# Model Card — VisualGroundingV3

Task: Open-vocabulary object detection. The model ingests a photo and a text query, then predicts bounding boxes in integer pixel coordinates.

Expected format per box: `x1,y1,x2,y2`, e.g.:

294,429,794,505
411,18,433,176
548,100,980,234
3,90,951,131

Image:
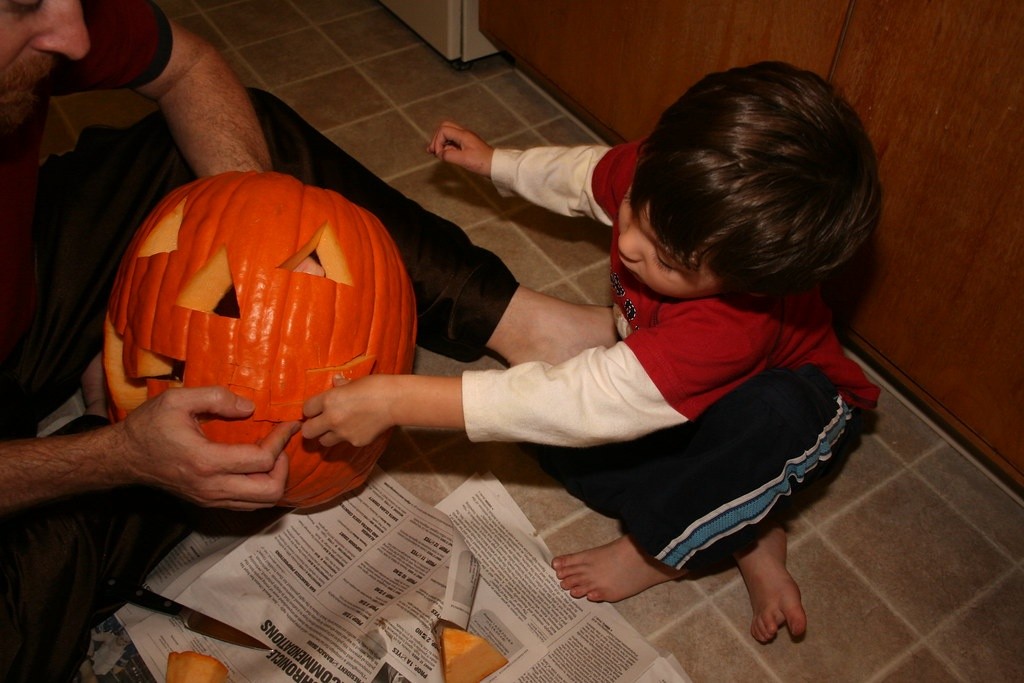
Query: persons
0,0,623,683
301,60,882,645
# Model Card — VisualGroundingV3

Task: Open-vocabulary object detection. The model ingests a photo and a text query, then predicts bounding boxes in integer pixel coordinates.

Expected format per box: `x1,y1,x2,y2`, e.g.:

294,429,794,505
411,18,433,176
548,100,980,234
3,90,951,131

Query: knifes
128,586,275,653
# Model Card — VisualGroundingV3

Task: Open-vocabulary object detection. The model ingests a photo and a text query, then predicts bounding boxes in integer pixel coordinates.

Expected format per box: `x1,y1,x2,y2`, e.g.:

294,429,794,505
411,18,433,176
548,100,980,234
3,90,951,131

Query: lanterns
102,169,419,512
